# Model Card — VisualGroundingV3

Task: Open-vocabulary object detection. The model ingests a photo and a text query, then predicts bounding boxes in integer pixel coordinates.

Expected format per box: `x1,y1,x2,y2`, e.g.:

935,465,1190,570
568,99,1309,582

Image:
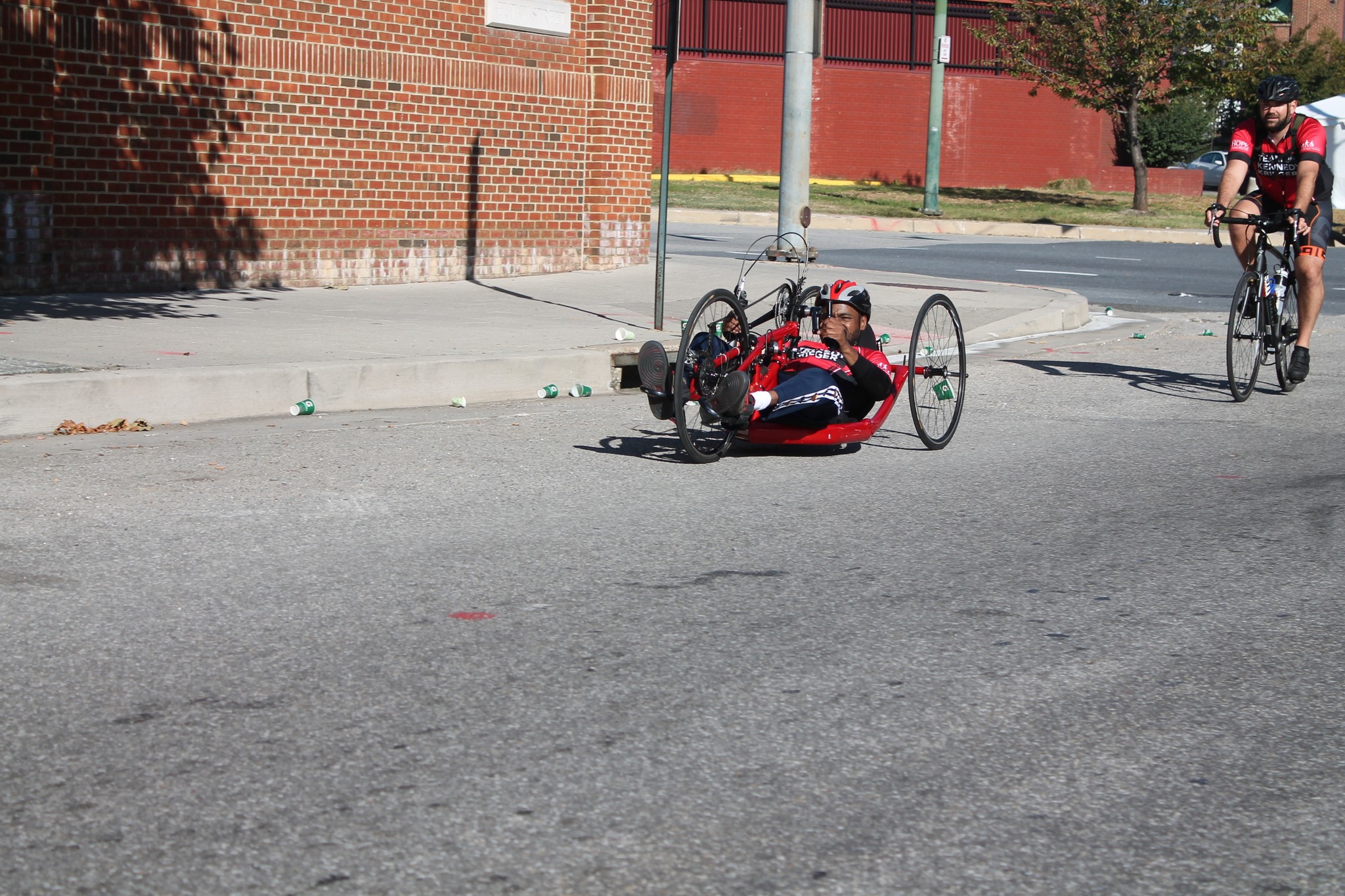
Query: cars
1167,151,1259,195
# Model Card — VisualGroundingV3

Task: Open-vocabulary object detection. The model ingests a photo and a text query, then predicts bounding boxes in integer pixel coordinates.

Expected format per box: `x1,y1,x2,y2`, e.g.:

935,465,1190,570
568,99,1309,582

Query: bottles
1270,263,1291,324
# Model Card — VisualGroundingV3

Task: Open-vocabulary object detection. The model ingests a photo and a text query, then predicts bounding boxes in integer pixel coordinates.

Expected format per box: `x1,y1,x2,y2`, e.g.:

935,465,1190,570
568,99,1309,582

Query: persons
1205,75,1334,380
637,279,893,425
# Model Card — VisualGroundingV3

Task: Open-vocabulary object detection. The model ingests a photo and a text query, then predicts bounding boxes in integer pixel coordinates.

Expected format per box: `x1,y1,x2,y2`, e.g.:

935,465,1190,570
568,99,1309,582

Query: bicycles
1207,204,1301,402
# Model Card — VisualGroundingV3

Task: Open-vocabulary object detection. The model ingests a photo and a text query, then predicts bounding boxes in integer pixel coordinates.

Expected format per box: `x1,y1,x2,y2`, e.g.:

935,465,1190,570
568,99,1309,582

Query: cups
1202,329,1215,335
289,398,315,416
452,396,467,408
536,384,558,399
1131,332,1147,339
715,321,723,338
876,334,890,345
572,383,593,398
933,378,955,401
681,319,688,334
920,347,935,355
1105,306,1114,316
615,328,634,341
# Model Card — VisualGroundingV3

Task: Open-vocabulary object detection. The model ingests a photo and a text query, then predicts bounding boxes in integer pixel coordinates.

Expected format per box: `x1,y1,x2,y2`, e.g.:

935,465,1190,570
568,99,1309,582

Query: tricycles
639,231,968,463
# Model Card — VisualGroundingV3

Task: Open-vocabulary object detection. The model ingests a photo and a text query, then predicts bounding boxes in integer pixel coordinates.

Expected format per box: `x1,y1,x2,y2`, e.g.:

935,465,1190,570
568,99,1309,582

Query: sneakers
1287,349,1310,380
1236,285,1258,318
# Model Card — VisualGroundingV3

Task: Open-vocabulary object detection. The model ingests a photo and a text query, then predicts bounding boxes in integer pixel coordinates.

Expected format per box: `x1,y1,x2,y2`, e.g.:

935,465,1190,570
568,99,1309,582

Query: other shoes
699,371,755,424
638,340,690,420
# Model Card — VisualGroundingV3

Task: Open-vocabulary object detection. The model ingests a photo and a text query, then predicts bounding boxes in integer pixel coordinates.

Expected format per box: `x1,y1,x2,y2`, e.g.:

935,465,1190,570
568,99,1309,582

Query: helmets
1256,76,1299,102
813,279,871,324
